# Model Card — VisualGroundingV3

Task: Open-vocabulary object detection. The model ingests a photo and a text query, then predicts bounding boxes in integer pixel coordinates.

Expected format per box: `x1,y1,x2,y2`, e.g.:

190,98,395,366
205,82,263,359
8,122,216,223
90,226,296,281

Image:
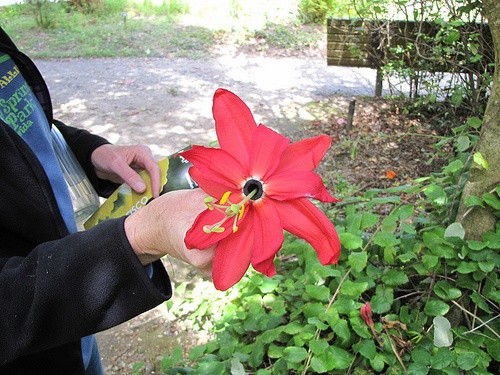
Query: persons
0,24,219,375
